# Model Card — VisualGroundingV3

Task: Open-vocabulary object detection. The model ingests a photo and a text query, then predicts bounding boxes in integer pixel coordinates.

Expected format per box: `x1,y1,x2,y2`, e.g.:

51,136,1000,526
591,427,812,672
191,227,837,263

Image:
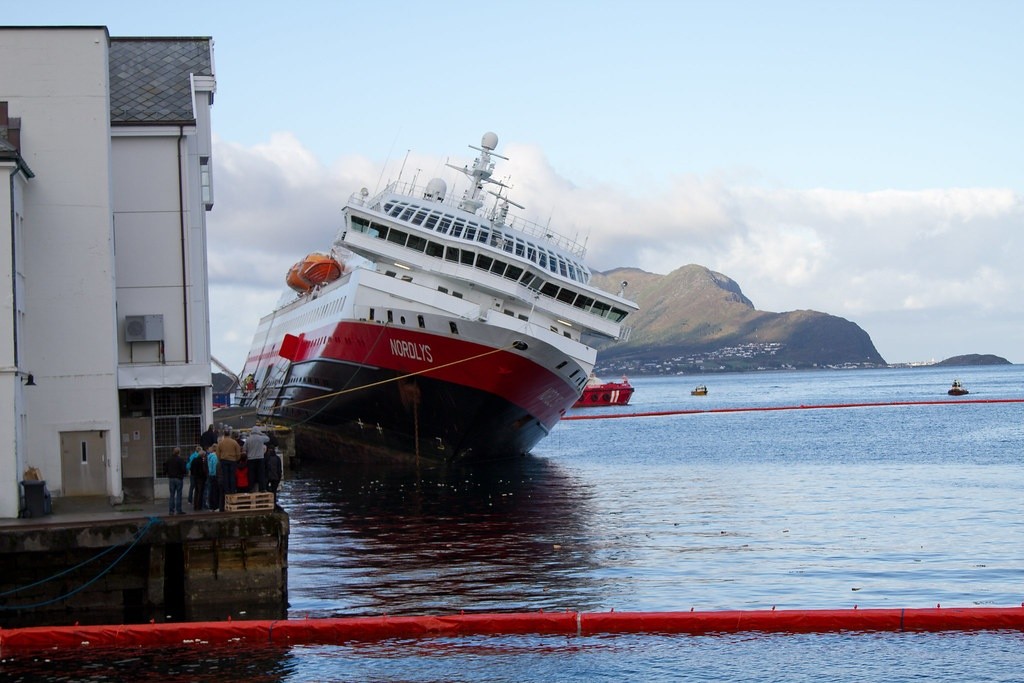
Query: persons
216,431,241,494
201,424,245,451
186,444,220,512
162,448,187,515
243,426,270,493
242,374,254,389
263,448,282,503
236,462,249,494
622,373,628,383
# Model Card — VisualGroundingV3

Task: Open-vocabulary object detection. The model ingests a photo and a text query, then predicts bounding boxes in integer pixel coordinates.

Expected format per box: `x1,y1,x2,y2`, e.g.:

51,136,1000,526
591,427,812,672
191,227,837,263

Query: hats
251,426,261,434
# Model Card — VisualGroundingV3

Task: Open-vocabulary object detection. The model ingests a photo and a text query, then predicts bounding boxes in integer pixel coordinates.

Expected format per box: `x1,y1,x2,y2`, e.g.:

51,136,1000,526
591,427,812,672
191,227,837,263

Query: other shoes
177,512,185,515
170,511,174,515
207,508,219,512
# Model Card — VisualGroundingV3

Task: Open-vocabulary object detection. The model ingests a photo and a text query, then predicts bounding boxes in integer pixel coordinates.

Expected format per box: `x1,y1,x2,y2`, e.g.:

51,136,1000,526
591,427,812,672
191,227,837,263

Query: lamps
21,374,36,386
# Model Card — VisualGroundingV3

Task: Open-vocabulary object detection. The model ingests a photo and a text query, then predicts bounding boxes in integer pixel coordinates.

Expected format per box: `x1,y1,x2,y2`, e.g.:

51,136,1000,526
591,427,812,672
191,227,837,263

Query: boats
690,385,708,395
297,254,343,286
571,374,634,407
285,262,319,293
230,130,640,478
947,380,970,395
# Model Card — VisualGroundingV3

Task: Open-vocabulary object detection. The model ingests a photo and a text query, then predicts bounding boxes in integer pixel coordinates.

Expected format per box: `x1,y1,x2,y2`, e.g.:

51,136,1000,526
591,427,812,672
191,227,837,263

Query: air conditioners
124,313,165,342
